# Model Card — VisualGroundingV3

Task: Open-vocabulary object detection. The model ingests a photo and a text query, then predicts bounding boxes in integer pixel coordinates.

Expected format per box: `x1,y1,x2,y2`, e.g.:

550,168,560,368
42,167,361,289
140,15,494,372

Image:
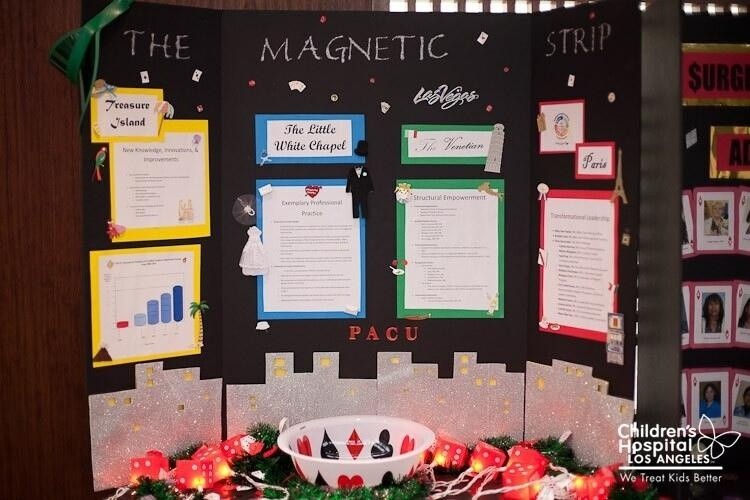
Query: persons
701,293,725,333
733,386,750,418
704,201,729,237
699,383,721,418
738,296,750,329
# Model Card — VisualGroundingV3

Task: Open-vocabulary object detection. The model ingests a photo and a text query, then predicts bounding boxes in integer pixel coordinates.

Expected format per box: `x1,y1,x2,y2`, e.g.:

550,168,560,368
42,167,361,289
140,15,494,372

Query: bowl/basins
273,413,438,491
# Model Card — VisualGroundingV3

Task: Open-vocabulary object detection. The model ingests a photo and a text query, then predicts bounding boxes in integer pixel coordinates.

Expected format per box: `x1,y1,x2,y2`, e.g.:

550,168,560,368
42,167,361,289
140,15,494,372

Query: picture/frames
536,99,586,156
